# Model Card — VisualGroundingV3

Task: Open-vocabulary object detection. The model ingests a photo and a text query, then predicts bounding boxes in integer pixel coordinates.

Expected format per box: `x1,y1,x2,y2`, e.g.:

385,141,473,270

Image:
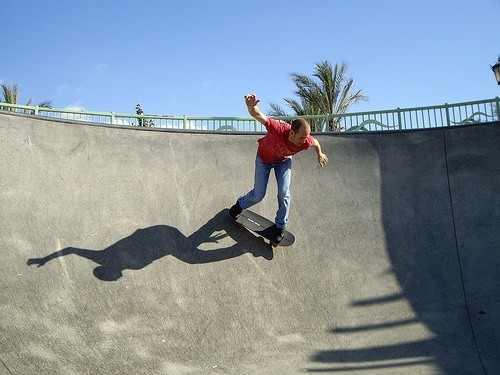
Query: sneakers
273,227,286,244
233,198,243,219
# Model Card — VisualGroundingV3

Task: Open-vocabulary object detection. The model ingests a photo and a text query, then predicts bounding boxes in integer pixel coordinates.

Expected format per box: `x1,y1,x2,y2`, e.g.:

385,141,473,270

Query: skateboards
231,209,295,247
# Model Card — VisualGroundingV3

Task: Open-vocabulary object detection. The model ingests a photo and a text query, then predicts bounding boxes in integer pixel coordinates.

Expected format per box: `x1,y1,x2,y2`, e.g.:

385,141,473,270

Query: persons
229,92,329,244
